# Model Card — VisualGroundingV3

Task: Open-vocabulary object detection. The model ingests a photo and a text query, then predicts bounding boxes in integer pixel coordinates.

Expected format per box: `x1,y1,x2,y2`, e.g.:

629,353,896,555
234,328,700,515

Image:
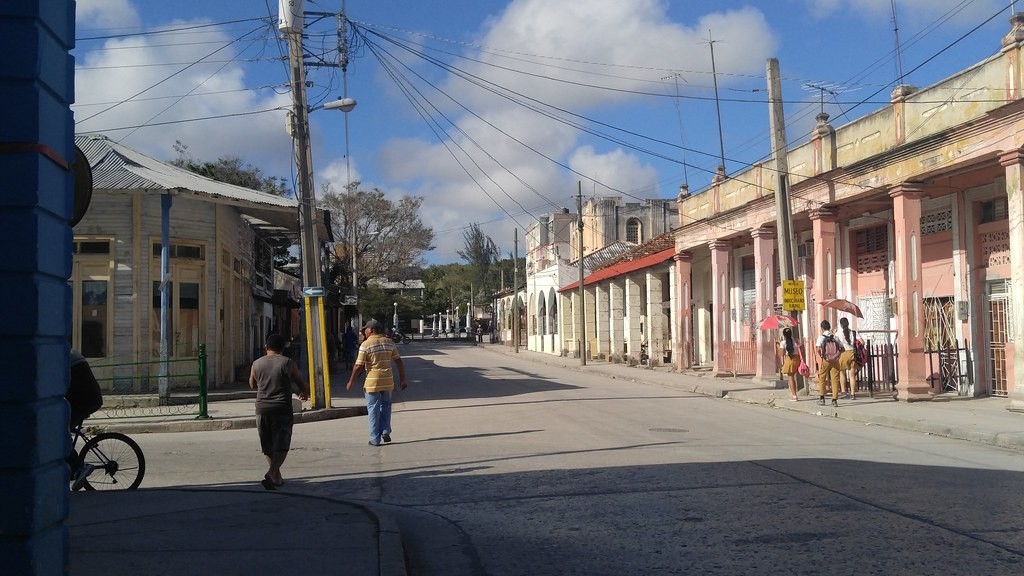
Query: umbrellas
818,296,864,328
751,314,800,330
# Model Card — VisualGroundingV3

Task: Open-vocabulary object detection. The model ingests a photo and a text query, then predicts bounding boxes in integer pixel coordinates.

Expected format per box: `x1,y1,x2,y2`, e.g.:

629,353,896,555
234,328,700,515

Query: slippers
274,478,283,486
261,478,277,490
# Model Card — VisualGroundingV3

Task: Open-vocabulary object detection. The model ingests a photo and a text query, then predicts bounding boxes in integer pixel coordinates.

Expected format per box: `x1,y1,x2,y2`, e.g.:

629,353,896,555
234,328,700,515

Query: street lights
293,98,356,410
351,233,380,331
432,314,436,330
393,302,398,329
466,303,471,332
446,309,450,331
439,312,443,331
455,307,459,332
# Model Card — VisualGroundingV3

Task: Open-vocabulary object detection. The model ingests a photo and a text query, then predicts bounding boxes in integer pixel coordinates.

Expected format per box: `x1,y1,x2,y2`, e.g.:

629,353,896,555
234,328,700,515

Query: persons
346,321,406,446
777,328,804,401
489,322,494,343
69,350,95,491
326,326,358,374
833,317,865,400
477,325,483,343
386,325,400,338
249,334,308,489
816,320,845,406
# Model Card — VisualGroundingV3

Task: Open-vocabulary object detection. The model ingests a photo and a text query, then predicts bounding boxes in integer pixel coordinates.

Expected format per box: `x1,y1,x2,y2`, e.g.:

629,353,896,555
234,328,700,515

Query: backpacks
823,334,841,362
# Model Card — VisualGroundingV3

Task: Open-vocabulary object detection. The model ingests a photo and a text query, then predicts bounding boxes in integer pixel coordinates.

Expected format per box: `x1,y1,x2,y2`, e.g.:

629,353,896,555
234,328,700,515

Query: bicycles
70,417,145,493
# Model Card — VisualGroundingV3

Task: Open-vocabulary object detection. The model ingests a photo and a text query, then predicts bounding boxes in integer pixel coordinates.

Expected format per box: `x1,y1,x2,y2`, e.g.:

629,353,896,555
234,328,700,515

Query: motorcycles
386,332,410,344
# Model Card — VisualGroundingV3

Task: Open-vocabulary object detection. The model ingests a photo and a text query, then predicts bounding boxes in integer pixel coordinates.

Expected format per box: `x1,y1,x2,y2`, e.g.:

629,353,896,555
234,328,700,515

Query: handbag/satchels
852,330,867,366
798,362,809,377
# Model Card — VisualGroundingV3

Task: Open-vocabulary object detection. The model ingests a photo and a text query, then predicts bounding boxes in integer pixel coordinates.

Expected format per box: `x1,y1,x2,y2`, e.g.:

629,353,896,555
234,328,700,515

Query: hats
360,320,381,332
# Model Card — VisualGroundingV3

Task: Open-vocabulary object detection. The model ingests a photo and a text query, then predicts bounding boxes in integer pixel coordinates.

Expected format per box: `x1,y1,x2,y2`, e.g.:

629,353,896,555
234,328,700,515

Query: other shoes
72,463,94,491
790,396,798,401
368,440,378,445
851,395,855,401
831,400,837,407
818,399,824,405
381,434,390,442
838,392,847,399
